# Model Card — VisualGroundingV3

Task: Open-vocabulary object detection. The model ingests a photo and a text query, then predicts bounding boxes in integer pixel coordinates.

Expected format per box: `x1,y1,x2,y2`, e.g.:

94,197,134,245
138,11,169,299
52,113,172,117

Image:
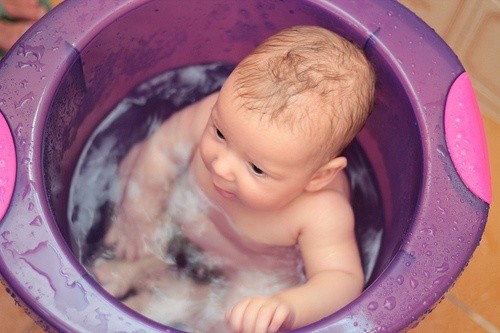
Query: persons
94,23,375,333
